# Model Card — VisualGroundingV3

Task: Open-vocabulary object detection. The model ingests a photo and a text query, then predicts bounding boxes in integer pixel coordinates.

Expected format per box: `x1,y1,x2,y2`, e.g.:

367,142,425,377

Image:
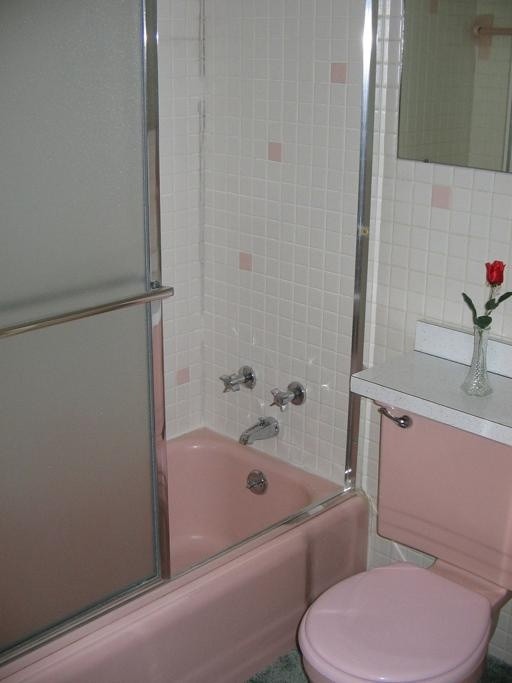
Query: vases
461,325,492,396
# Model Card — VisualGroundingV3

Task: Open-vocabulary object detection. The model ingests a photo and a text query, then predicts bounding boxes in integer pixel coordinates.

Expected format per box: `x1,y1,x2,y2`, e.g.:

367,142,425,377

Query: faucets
239,417,280,445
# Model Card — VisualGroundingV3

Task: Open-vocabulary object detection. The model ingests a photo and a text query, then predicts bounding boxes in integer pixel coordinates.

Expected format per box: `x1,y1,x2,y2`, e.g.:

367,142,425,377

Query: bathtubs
0,427,369,683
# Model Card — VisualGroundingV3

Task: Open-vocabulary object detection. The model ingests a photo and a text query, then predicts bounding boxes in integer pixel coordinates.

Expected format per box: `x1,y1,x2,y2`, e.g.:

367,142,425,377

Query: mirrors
398,2,511,172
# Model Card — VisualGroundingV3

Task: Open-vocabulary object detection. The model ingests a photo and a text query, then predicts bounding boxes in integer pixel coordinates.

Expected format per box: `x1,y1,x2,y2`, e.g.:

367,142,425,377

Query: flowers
458,259,512,397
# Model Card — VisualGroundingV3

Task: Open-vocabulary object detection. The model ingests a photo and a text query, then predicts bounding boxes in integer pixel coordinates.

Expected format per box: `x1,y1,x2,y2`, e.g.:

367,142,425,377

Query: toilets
296,403,512,682
